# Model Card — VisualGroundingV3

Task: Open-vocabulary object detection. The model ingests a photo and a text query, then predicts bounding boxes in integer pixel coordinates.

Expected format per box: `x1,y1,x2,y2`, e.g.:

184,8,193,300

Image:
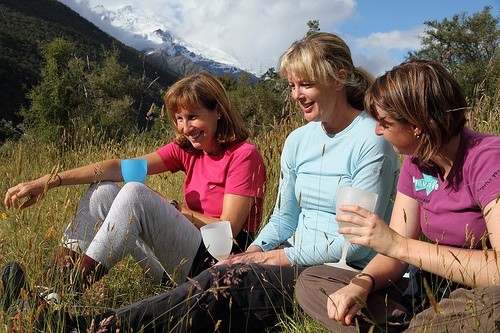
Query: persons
293,60,500,333
0,69,267,314
31,33,400,333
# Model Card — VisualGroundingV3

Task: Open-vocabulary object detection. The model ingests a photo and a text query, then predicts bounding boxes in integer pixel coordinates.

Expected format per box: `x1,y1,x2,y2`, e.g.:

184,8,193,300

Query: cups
120,158,148,183
200,220,232,261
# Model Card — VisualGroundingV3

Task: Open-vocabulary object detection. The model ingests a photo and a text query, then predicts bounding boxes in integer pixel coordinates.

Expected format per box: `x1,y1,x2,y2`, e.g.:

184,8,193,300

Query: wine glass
323,185,378,272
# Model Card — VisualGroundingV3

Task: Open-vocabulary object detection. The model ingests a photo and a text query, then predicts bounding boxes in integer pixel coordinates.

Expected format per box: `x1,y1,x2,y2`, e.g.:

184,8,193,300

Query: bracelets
355,273,375,292
50,171,61,186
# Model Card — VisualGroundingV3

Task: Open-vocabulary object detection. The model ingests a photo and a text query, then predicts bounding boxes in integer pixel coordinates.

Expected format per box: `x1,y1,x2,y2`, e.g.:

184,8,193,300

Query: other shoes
2,262,25,329
30,294,75,333
